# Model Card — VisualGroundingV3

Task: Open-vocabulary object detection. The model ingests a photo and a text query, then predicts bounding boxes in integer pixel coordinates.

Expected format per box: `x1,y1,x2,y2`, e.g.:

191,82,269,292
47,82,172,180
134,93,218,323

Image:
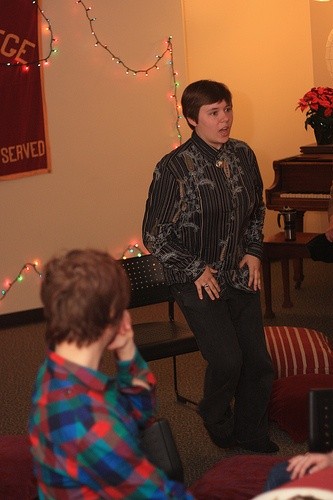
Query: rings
204,283,209,288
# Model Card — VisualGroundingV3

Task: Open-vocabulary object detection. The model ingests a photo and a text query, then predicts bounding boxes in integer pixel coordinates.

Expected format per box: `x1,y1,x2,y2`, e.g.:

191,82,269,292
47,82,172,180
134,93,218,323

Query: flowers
296,85,333,117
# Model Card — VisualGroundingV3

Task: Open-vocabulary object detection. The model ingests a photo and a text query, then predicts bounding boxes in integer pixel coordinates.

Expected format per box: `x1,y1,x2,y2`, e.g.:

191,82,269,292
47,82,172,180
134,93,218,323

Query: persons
267,452,333,492
28,250,193,500
143,80,279,454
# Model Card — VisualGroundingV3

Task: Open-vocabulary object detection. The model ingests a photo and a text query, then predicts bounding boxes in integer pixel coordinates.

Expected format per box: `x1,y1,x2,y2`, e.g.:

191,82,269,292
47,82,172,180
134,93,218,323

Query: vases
305,114,333,146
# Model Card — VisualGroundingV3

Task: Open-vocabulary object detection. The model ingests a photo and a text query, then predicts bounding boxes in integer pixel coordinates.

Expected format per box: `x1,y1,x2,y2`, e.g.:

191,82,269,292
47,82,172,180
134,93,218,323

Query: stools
262,232,333,319
260,326,333,446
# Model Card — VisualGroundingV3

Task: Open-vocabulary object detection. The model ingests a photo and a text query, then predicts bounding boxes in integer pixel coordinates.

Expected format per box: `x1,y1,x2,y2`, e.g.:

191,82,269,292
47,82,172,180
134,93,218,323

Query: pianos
265,141,333,311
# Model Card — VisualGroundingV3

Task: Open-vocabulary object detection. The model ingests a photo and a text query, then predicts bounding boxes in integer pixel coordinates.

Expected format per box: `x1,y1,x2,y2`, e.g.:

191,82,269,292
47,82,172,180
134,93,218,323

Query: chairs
114,255,214,408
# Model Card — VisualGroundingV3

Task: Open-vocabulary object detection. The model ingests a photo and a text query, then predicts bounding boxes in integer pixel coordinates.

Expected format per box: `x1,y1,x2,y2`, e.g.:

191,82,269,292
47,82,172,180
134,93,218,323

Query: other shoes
200,400,240,448
236,435,279,453
